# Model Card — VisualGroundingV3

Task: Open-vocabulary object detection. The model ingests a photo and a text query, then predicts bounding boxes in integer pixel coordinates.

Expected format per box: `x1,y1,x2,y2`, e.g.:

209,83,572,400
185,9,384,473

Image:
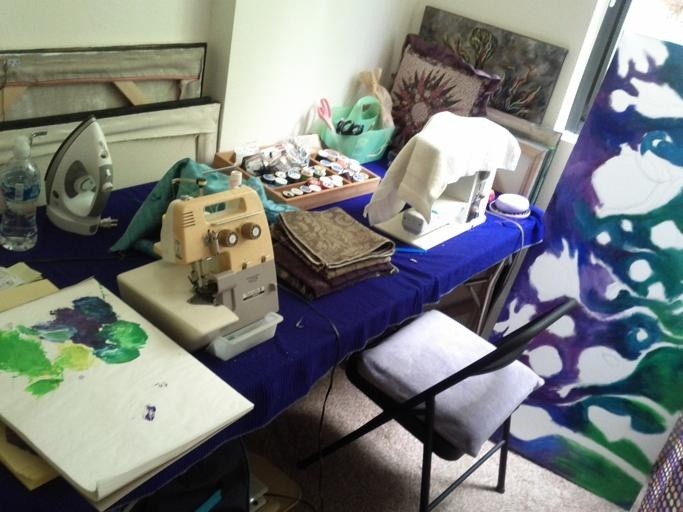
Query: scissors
317,98,363,135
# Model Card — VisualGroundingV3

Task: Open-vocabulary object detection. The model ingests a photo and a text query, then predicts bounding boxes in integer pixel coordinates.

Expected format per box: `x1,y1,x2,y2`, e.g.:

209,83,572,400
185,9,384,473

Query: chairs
286,297,580,512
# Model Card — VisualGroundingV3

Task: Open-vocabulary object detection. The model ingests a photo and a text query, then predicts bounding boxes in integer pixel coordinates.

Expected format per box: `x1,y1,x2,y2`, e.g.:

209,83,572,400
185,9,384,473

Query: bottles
1,136,40,252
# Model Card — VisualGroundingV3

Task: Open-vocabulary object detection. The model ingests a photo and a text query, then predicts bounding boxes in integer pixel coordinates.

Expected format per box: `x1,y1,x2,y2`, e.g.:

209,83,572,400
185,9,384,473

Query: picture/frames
485,106,563,205
2,43,220,208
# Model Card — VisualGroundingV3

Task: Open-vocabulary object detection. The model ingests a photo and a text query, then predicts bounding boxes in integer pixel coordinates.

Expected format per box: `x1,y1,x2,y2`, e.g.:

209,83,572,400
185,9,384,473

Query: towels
268,206,400,303
362,110,523,227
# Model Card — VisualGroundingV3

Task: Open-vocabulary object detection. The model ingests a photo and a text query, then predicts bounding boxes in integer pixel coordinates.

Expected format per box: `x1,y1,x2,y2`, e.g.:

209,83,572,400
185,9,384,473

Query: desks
0,161,547,512
